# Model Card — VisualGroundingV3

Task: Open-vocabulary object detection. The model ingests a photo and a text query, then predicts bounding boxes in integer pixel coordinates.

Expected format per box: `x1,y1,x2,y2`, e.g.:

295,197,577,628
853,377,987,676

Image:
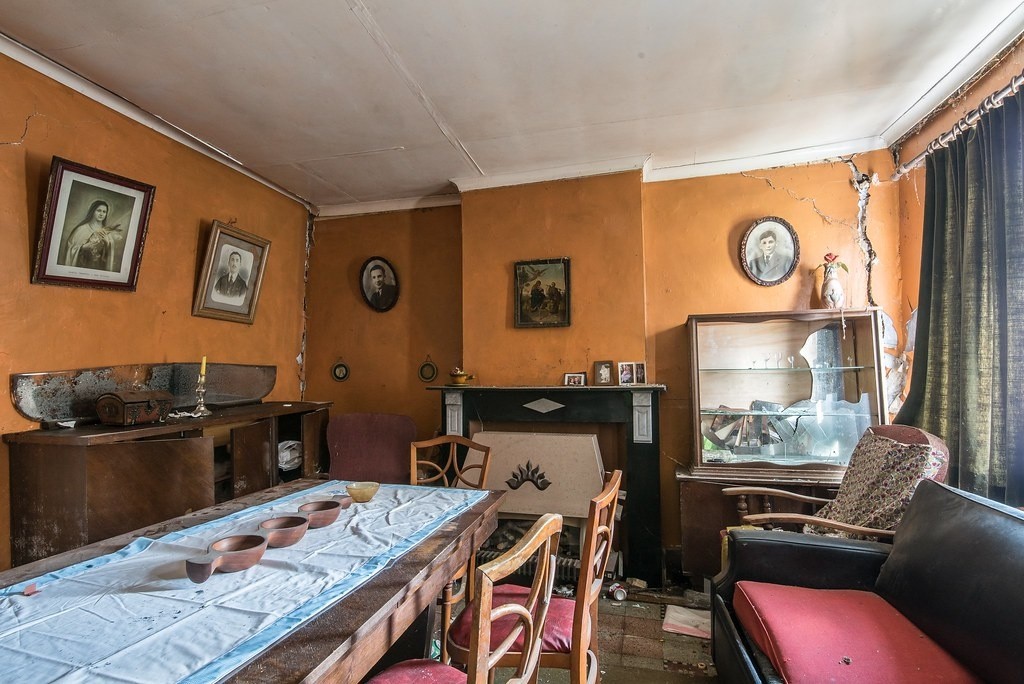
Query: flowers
811,253,849,276
449,367,467,374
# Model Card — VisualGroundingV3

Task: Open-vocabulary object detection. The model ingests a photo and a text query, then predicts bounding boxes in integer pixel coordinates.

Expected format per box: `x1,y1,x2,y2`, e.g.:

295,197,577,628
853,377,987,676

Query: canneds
609,583,627,601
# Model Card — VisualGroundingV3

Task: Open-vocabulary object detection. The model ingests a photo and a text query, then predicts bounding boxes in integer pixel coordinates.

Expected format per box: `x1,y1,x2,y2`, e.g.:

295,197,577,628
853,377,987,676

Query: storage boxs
603,551,623,582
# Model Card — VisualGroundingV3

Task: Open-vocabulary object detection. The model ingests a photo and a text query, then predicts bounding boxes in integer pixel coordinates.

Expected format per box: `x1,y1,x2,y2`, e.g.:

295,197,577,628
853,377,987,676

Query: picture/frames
192,219,272,325
30,155,156,293
740,216,800,287
564,372,586,386
513,256,572,329
635,362,647,385
359,256,399,313
617,362,637,386
594,360,615,386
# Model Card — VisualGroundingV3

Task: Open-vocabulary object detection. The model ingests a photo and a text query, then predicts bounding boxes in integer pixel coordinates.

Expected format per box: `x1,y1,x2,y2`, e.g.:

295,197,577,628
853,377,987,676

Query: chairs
410,434,492,666
364,512,563,684
713,425,949,584
446,469,623,684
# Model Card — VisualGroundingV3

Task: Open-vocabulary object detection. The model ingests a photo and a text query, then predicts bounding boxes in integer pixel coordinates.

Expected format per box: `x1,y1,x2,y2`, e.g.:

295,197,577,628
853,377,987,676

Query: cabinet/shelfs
674,463,842,594
2,401,334,570
684,306,889,482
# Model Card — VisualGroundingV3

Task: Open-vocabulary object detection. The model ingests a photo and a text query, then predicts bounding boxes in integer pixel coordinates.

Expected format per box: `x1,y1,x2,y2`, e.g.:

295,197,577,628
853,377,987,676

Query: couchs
710,478,1024,684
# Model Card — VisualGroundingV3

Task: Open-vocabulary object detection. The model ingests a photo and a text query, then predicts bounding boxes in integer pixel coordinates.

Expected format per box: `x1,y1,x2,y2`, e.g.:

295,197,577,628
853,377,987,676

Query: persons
215,252,247,298
369,265,396,308
569,378,581,384
65,200,114,272
749,230,793,281
600,365,607,379
621,365,631,382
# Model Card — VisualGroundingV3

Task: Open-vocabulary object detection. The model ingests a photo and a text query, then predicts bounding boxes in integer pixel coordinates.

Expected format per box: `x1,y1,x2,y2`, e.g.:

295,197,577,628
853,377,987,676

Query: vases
820,266,846,310
450,374,467,384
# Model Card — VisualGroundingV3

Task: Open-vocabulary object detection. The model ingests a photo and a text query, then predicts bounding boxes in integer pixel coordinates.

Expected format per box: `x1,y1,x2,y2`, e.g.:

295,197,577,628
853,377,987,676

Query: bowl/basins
346,482,379,503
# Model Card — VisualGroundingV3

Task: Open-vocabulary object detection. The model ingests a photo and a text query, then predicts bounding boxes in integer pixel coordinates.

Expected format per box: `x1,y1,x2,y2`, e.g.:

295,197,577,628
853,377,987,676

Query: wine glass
775,352,782,368
762,353,770,368
787,356,795,368
750,356,758,369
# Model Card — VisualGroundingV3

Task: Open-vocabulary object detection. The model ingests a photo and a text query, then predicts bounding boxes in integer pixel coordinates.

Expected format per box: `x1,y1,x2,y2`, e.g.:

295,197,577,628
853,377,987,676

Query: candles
200,355,206,375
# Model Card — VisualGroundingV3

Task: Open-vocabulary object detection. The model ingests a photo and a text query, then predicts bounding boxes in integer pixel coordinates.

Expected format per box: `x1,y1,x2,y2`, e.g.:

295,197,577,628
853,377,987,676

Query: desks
0,478,508,684
